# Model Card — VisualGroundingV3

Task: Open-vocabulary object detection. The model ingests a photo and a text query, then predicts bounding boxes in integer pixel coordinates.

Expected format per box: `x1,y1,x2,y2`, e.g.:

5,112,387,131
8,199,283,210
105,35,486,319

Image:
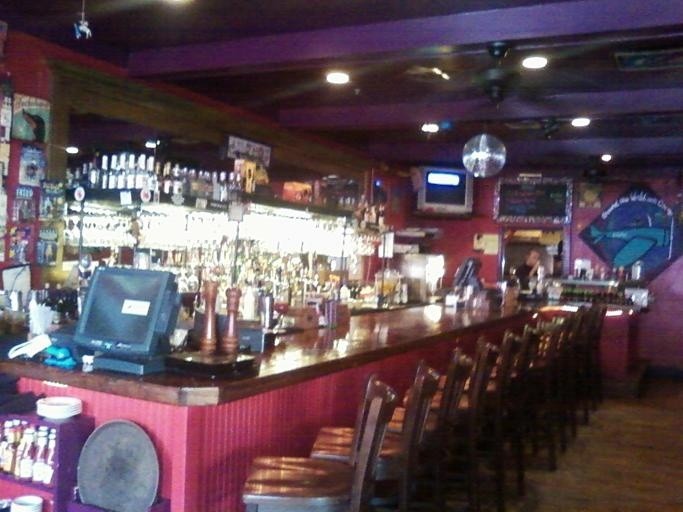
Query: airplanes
589,224,673,269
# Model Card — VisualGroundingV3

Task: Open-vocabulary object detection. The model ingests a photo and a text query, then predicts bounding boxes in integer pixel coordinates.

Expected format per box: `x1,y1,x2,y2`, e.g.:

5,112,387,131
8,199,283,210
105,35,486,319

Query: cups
10,494,42,512
2,308,26,336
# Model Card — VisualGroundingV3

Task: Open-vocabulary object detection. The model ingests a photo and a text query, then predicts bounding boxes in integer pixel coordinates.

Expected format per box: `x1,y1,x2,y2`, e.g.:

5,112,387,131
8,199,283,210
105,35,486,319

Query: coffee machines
397,226,444,302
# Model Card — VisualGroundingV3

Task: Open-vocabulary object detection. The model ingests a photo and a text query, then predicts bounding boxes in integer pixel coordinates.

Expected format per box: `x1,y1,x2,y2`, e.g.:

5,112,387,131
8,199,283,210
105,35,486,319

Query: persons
509,247,540,290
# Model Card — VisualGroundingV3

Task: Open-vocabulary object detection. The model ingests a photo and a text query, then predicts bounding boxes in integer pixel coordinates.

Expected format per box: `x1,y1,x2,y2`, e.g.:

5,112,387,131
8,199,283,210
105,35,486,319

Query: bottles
17,200,30,222
88,153,242,204
67,236,409,355
313,175,384,226
508,259,643,300
51,284,64,322
40,283,52,308
0,419,56,487
0,289,22,311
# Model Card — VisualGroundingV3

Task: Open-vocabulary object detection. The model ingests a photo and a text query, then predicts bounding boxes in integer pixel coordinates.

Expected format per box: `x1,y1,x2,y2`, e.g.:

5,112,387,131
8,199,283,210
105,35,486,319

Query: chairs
385,350,469,501
242,374,397,511
315,358,441,510
403,337,498,507
431,301,609,495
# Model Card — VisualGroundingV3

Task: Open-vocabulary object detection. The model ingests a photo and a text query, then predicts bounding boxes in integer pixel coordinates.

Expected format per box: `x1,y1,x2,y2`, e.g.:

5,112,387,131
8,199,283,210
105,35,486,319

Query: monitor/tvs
74,264,183,378
453,256,487,294
416,164,473,215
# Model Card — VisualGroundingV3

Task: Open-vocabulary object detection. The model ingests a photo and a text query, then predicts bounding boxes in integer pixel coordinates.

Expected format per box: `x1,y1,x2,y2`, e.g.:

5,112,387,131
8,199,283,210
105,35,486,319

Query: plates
34,396,83,420
